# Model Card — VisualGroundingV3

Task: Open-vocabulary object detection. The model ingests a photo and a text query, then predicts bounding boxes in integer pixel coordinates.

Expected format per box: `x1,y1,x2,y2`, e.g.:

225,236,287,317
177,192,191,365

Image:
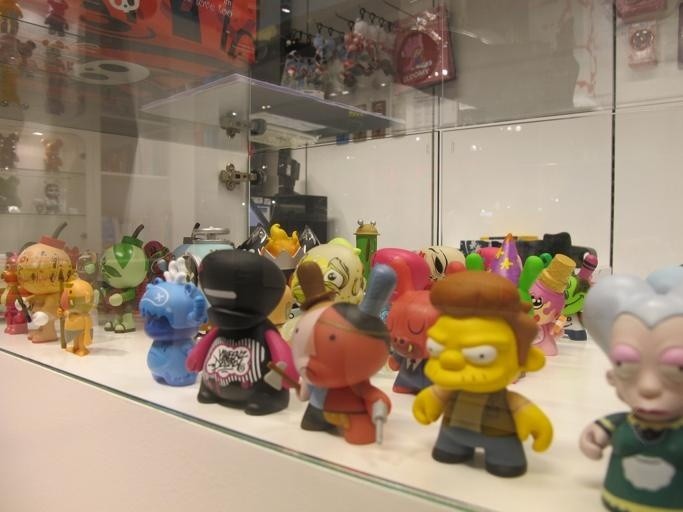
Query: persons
1,222,683,512
0,0,70,216
0,217,681,512
283,10,407,97
